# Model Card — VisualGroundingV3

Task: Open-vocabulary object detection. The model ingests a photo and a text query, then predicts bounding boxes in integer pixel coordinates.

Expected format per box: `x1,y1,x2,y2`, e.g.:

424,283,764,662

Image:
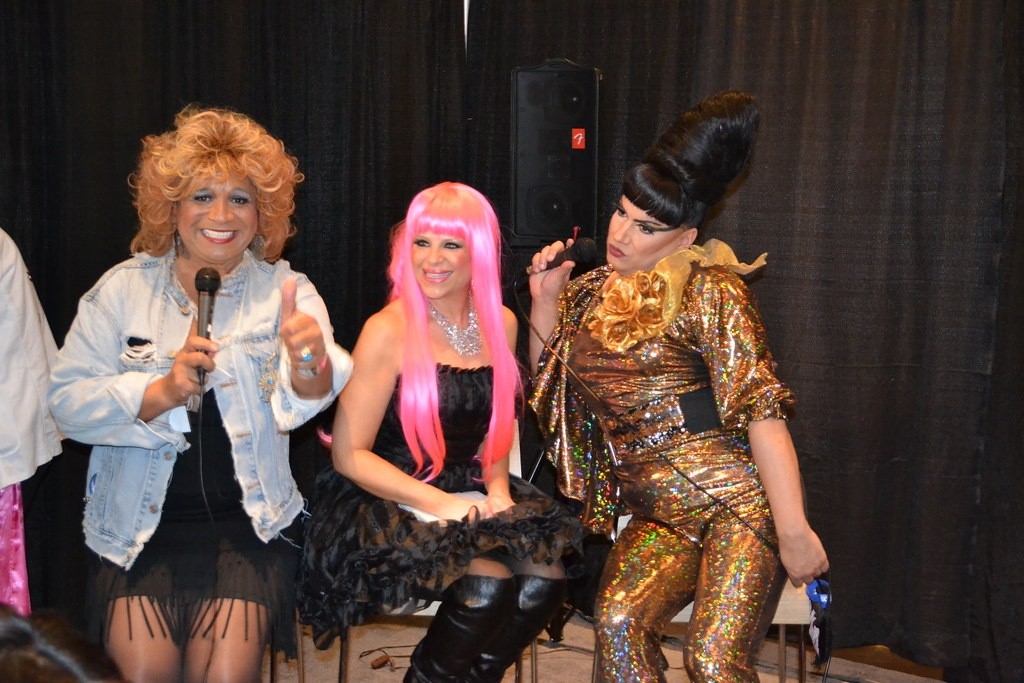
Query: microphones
196,267,221,386
516,237,597,287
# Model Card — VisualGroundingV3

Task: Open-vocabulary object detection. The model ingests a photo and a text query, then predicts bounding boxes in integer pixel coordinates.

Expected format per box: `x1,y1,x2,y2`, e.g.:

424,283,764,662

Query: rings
301,347,313,362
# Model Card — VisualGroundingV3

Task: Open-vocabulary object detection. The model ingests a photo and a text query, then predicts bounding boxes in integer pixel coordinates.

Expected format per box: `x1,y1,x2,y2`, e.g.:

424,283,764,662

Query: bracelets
296,351,329,378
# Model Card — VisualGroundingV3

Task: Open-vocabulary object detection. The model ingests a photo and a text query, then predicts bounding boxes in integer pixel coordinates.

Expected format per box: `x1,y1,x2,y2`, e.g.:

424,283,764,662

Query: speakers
507,57,603,247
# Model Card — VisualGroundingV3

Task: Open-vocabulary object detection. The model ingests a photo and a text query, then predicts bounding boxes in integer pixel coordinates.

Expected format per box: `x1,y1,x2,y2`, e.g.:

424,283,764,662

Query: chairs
268,502,818,683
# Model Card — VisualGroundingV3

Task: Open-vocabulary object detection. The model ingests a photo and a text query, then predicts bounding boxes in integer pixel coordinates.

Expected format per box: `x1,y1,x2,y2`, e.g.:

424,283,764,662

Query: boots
403,573,570,683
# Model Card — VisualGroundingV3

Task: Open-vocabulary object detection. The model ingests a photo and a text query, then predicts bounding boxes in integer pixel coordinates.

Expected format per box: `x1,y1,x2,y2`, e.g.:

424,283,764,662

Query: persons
529,90,830,683
0,108,355,683
305,180,584,683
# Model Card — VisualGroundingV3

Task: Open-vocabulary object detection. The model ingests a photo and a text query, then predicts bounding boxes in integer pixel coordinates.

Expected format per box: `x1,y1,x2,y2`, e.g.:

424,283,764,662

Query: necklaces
426,291,484,357
